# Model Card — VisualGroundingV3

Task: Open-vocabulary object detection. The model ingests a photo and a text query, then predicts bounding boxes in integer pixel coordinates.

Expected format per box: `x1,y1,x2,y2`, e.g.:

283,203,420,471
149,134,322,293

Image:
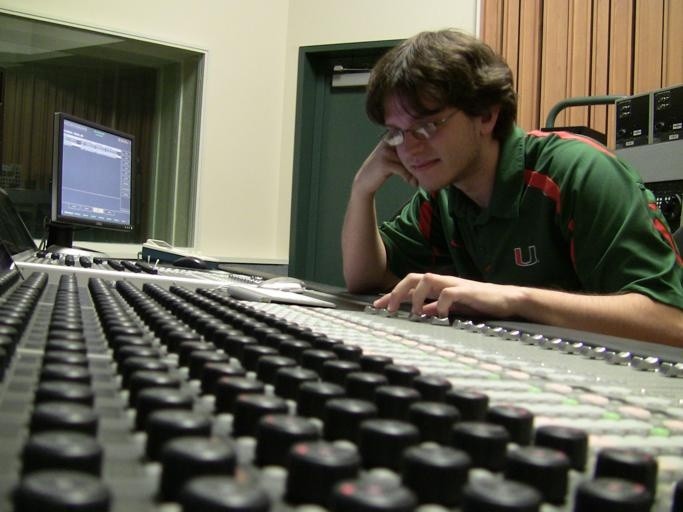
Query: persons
339,29,683,350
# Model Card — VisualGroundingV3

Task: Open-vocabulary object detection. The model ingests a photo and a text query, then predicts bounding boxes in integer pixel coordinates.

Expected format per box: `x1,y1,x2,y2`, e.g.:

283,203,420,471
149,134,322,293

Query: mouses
176,257,208,269
259,277,305,294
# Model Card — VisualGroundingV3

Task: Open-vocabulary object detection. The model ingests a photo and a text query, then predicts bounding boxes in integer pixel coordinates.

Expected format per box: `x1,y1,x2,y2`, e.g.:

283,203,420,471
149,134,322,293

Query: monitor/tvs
50,111,137,257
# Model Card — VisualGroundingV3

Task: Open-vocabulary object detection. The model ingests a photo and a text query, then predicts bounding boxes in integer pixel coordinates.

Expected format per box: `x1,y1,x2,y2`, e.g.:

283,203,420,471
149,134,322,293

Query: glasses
383,109,462,146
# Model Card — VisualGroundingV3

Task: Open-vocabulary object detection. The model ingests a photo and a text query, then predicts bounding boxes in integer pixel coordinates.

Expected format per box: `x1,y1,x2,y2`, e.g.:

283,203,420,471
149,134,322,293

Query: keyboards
230,285,334,306
